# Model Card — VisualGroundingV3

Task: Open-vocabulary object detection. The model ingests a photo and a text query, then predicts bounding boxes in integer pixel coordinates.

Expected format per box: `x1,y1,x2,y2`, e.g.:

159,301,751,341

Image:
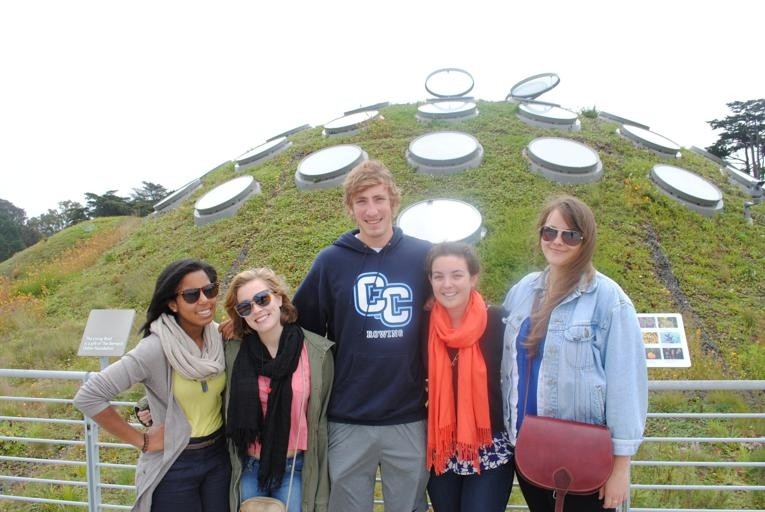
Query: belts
185,436,221,450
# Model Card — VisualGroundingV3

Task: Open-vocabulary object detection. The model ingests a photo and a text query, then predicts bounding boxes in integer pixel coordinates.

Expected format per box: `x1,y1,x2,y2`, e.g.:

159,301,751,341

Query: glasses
233,290,273,317
541,225,584,246
178,283,218,303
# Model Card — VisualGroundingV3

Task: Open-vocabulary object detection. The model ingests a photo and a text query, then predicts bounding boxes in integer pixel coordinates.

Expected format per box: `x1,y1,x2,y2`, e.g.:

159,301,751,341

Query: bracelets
141,432,148,453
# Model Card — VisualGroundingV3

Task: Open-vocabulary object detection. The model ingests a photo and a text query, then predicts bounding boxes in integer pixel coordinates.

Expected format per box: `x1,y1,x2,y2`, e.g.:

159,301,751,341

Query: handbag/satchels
516,414,615,497
238,497,287,512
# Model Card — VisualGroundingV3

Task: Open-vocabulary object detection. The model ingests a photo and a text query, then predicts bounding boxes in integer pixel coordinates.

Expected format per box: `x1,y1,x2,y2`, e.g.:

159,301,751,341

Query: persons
416,195,649,512
217,161,435,511
74,261,233,510
420,241,514,511
134,269,335,511
